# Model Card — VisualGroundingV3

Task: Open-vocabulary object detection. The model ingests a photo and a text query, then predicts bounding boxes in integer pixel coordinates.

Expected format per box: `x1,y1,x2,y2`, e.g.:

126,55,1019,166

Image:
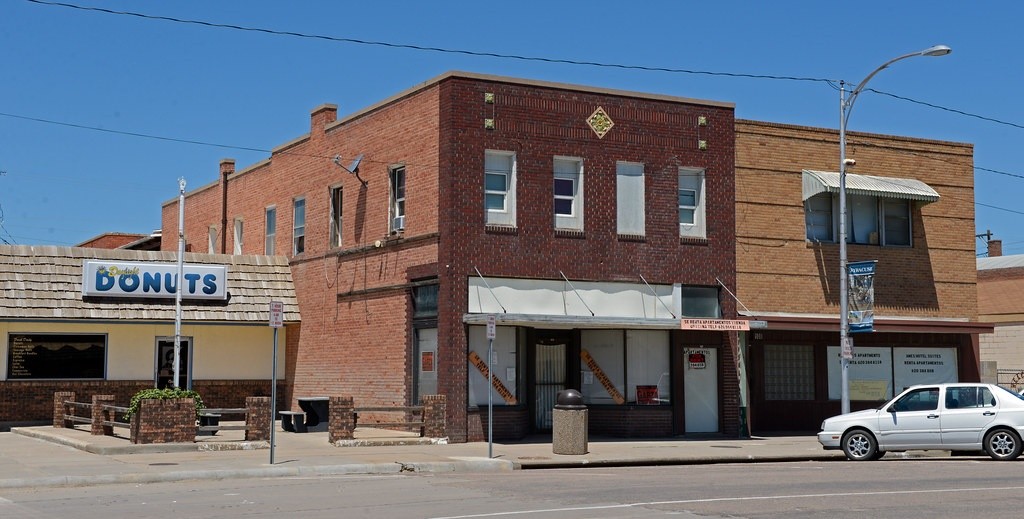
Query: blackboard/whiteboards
5,332,108,381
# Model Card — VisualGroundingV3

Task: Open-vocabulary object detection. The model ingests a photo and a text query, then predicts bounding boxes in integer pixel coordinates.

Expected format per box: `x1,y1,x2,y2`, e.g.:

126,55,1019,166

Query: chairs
949,398,957,408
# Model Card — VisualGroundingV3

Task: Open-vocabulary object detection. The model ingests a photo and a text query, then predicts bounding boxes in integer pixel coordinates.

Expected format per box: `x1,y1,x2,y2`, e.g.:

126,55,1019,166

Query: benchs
196,412,221,436
278,411,308,434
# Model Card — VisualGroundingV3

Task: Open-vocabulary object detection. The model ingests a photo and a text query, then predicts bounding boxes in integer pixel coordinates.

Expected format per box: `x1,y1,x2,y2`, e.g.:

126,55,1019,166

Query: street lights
840,44,952,413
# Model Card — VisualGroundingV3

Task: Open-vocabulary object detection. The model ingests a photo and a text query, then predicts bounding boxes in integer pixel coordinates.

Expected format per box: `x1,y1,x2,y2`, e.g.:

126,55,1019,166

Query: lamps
376,237,404,248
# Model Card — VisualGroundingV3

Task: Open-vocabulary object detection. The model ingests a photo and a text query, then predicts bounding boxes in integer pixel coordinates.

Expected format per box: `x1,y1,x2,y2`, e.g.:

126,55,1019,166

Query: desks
290,396,331,430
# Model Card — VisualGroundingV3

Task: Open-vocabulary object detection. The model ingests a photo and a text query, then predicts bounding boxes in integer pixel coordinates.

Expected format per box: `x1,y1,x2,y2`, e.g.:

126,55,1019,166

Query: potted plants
122,387,204,446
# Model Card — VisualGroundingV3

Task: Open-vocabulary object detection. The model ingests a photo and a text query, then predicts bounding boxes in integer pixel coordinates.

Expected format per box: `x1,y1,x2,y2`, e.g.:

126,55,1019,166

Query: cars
817,383,1024,461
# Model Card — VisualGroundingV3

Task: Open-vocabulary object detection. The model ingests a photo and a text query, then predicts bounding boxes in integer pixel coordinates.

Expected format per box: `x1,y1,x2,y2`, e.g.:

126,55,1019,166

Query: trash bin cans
552,389,588,454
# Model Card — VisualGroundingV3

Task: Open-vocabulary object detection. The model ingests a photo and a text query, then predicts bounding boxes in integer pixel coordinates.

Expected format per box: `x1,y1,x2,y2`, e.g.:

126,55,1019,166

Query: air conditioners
394,215,406,231
